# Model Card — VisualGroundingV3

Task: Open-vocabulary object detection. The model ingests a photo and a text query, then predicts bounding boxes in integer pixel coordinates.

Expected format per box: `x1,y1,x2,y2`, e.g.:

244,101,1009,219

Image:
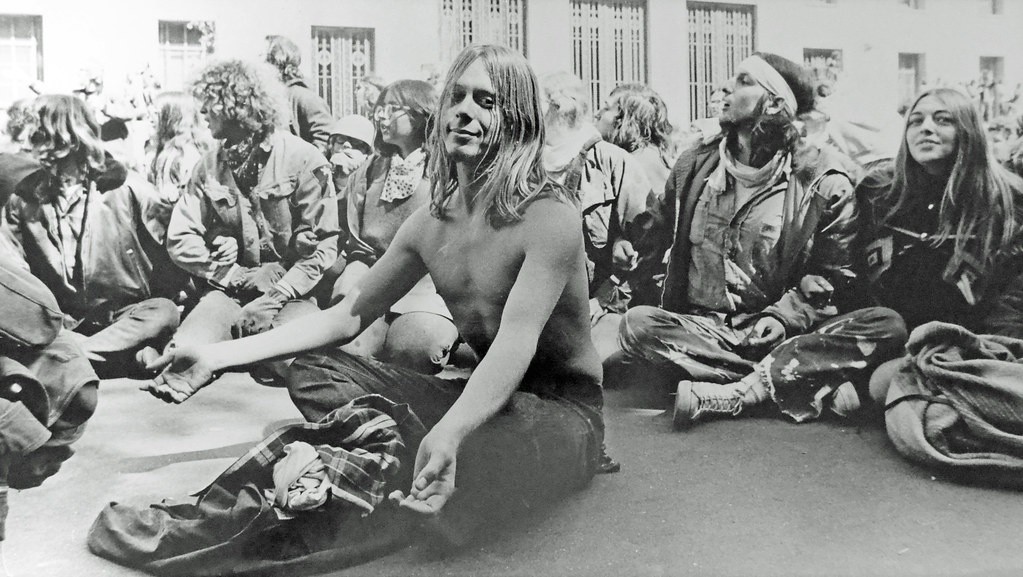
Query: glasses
335,135,363,147
373,104,418,122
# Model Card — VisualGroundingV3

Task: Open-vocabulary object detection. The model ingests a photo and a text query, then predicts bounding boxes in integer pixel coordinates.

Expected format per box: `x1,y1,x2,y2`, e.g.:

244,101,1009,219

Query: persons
938,70,1023,178
257,35,336,159
540,71,663,387
0,75,205,541
618,51,909,428
139,42,603,561
354,74,389,119
855,88,1023,407
689,85,736,145
594,83,681,204
330,114,376,196
331,79,466,375
161,57,338,384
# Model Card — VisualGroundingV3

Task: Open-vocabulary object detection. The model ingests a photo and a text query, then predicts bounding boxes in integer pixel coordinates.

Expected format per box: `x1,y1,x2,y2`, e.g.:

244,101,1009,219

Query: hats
328,115,375,153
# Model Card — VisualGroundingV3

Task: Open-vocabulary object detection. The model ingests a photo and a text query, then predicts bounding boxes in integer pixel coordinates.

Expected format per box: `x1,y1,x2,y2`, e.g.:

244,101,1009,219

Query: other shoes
673,377,747,424
828,381,864,420
